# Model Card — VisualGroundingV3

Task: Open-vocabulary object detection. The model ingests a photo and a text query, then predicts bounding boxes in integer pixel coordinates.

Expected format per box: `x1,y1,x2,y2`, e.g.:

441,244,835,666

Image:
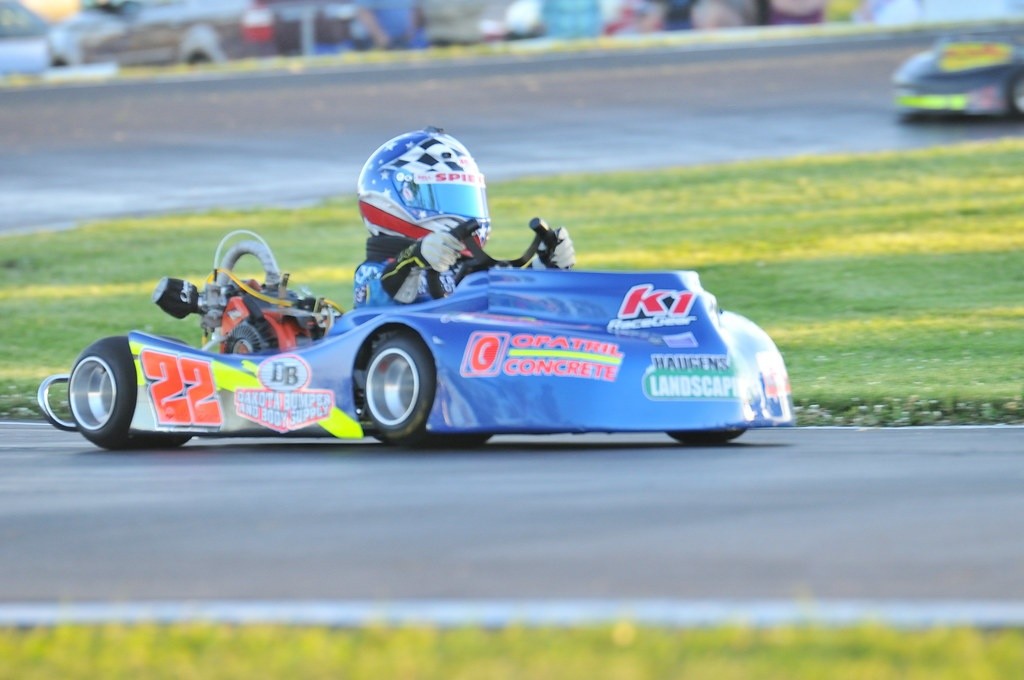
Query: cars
68,0,367,66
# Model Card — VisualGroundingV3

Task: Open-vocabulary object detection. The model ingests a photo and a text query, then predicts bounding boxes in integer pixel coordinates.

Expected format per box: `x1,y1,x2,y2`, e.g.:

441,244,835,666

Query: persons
351,127,577,327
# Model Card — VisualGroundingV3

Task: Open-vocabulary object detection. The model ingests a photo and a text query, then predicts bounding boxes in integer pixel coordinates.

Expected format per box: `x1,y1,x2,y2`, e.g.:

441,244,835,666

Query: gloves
420,232,466,273
532,227,575,270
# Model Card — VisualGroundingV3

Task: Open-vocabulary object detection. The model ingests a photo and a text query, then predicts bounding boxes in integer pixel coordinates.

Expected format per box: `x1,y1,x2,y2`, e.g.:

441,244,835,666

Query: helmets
357,126,490,258
